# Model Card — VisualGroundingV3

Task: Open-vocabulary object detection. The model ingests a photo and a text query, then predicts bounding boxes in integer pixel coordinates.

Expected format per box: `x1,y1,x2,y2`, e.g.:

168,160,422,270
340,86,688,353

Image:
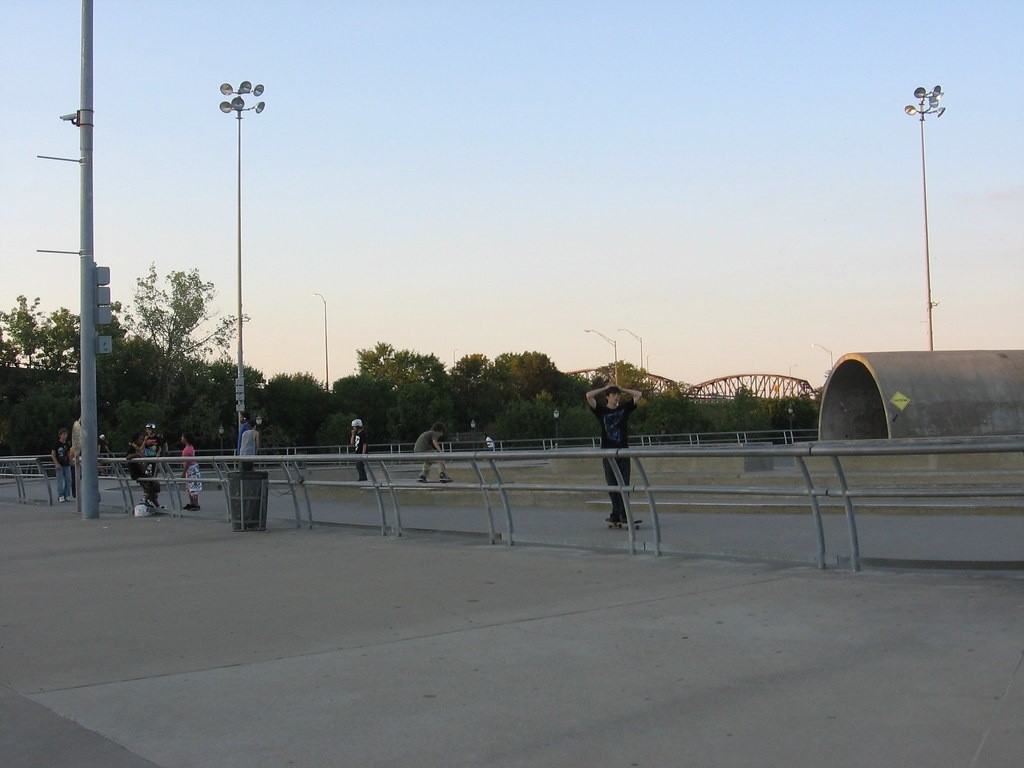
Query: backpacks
148,434,168,456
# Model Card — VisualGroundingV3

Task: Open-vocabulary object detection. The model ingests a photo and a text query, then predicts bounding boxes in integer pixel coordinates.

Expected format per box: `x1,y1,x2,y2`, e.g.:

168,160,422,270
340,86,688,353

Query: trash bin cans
228,471,269,531
294,450,307,469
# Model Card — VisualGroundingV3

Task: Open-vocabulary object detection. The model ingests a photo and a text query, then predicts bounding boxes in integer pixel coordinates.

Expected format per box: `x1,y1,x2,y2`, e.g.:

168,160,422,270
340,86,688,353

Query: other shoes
609,514,621,522
358,477,367,481
190,504,200,511
439,472,453,481
183,504,191,509
622,514,627,523
416,475,426,482
65,495,76,501
59,496,65,502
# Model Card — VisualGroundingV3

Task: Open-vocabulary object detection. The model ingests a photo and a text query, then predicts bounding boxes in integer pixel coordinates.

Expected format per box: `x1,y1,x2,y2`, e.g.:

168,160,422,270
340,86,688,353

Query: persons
51,428,77,502
350,419,367,481
239,420,260,472
181,432,203,511
483,432,495,448
97,434,110,458
414,422,454,482
586,383,642,523
127,433,161,507
141,422,168,478
237,417,250,455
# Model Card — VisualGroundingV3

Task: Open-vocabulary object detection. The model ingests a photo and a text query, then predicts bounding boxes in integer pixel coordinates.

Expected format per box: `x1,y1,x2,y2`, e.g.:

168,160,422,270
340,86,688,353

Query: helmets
351,419,363,426
145,422,158,429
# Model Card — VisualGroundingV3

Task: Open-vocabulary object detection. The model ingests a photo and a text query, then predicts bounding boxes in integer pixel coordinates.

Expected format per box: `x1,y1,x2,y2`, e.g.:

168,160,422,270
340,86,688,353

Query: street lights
453,346,460,369
903,83,947,351
646,353,654,377
217,79,267,442
584,329,618,385
312,292,330,392
617,328,643,370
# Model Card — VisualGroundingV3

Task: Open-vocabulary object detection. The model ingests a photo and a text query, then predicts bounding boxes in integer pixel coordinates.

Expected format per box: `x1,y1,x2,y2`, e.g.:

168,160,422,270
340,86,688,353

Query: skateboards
417,479,454,484
605,518,643,531
